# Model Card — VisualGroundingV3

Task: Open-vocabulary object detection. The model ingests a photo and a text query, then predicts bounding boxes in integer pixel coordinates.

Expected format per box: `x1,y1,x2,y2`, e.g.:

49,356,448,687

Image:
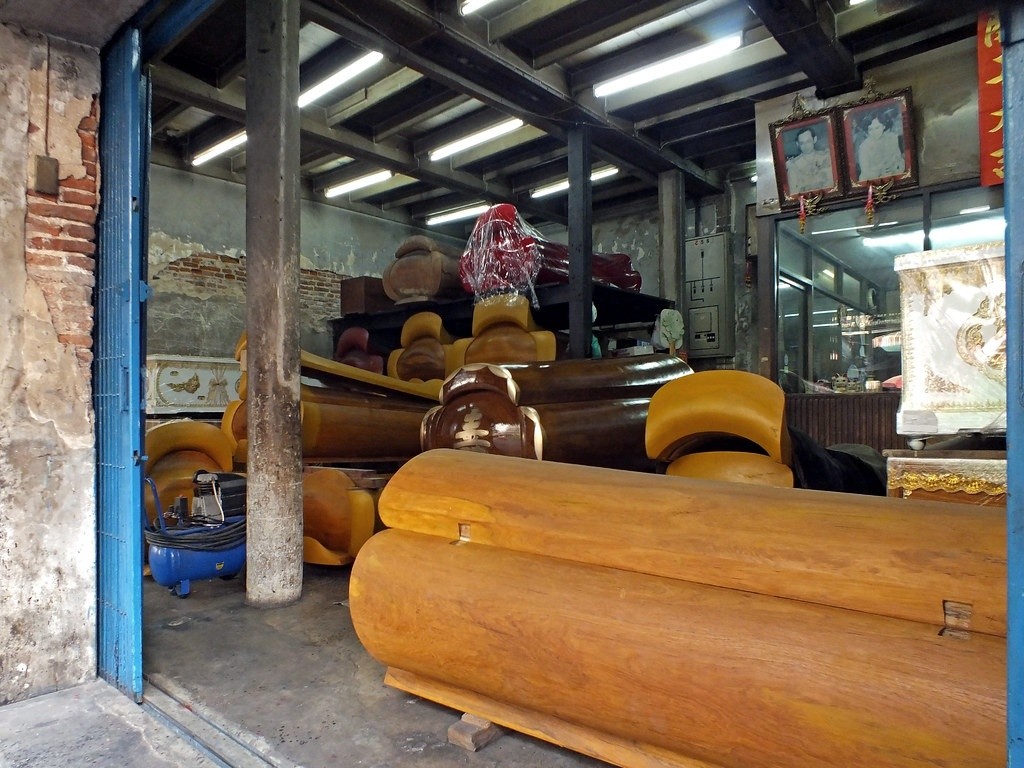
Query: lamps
173,0,747,226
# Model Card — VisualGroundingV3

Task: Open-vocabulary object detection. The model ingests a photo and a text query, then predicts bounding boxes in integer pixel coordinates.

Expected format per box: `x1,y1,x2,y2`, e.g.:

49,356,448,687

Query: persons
859,110,906,182
785,127,835,196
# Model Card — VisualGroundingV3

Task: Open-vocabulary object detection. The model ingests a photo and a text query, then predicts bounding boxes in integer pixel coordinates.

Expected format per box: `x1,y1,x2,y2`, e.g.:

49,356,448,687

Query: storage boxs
145,292,1007,768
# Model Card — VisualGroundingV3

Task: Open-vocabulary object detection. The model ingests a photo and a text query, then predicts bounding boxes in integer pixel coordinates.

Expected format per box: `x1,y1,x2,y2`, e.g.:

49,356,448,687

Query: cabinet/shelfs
327,278,677,353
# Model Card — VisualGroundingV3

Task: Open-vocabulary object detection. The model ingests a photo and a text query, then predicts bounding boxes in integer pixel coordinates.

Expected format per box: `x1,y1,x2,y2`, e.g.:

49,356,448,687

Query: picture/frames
770,76,919,210
